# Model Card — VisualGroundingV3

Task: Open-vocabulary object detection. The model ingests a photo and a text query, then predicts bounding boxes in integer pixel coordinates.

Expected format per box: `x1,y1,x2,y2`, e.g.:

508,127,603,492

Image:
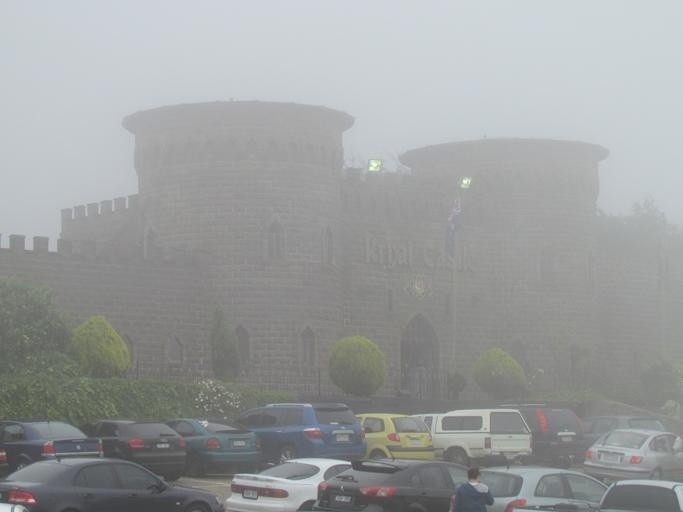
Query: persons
452,467,494,512
